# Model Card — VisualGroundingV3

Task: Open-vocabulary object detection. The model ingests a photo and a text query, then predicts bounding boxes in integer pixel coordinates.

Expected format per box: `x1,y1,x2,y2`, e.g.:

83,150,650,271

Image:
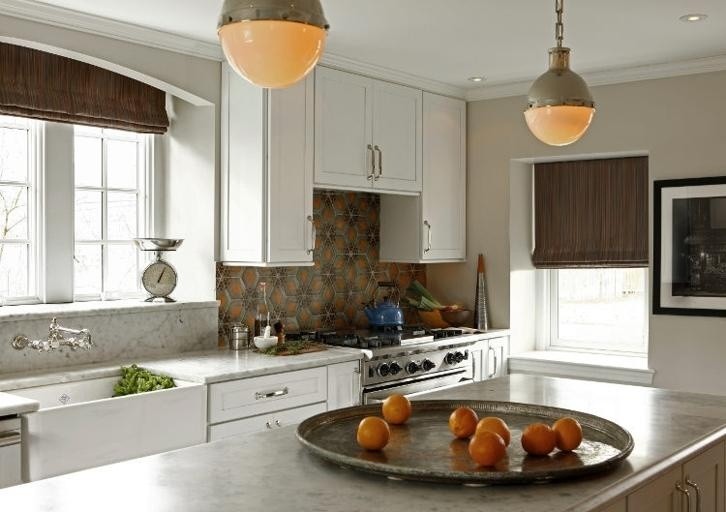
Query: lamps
216,1,329,89
522,0,597,146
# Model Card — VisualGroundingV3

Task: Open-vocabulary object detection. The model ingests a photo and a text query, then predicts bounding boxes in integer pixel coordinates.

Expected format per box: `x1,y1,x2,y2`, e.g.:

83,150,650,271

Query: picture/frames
652,177,726,317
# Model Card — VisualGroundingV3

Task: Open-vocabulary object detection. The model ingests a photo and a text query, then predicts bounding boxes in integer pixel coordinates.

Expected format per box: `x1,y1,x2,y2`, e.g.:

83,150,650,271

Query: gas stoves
294,324,473,387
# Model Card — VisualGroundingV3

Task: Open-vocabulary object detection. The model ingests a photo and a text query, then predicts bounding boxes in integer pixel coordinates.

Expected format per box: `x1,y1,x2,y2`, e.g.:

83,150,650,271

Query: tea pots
361,279,406,328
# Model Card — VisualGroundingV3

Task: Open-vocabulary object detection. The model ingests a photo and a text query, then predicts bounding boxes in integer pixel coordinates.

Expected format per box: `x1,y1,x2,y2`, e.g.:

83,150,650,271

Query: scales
133,237,184,302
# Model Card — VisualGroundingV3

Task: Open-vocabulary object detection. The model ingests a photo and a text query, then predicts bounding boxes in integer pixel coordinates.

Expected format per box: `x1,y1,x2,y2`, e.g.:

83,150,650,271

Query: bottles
253,280,271,335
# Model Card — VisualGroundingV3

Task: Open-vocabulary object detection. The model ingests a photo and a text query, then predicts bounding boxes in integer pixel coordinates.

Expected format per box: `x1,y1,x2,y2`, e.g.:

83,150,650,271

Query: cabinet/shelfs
120,345,364,442
1,389,39,486
380,92,466,264
316,65,423,197
222,62,315,269
628,420,726,510
488,328,513,379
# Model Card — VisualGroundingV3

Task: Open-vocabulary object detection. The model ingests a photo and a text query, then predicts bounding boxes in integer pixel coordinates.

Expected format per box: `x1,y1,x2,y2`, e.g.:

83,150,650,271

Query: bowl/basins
252,335,280,350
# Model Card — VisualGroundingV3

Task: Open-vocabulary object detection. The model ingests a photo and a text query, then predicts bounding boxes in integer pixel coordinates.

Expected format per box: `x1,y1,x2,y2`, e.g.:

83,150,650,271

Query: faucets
47,314,93,351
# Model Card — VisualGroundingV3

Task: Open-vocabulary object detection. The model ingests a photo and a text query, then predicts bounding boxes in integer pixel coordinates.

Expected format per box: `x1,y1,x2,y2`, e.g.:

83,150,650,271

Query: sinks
0,361,209,482
